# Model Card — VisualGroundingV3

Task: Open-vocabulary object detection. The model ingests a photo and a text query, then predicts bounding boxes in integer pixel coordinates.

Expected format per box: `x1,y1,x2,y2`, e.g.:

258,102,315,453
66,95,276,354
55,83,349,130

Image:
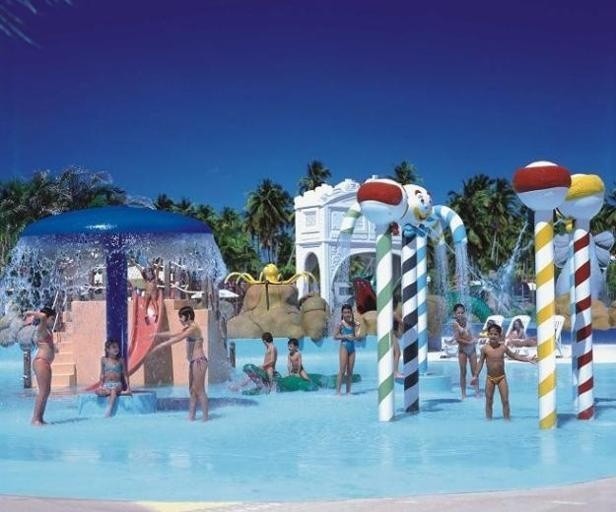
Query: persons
130,256,161,325
332,304,361,399
21,308,59,426
95,339,132,419
450,304,537,421
146,306,209,423
287,338,311,382
229,332,277,394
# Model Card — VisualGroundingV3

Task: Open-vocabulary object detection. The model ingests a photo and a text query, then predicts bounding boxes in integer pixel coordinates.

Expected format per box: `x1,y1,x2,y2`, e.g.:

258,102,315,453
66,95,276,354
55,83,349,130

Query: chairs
440,314,566,368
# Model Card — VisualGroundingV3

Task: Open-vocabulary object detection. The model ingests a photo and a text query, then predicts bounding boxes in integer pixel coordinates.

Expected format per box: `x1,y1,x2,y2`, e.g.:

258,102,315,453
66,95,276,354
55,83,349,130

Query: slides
83,285,163,391
